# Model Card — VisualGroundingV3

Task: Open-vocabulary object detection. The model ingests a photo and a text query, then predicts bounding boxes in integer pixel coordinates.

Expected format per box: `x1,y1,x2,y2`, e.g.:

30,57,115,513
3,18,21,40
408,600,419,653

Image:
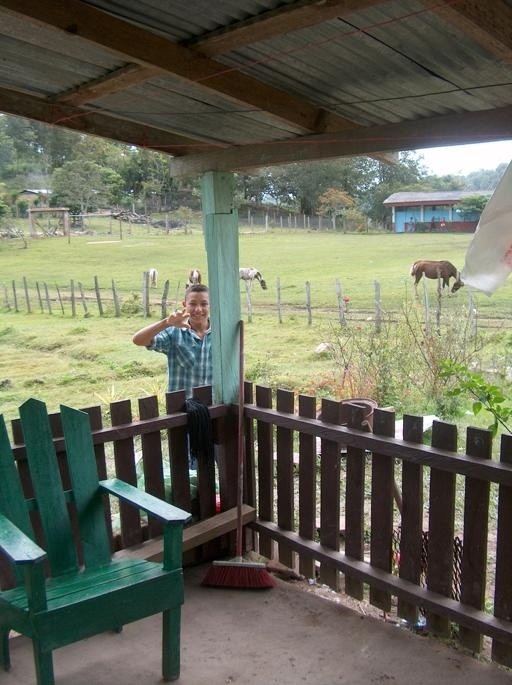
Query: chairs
0,399,193,685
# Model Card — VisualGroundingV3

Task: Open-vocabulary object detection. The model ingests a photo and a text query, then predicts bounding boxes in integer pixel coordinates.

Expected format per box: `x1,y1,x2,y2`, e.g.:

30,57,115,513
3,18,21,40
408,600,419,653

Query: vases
317,397,379,451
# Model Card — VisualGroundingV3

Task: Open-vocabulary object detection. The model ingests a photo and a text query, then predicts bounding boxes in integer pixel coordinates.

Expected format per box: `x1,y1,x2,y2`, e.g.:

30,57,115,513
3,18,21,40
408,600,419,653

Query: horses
239,268,268,290
150,268,158,289
411,260,464,301
185,269,201,290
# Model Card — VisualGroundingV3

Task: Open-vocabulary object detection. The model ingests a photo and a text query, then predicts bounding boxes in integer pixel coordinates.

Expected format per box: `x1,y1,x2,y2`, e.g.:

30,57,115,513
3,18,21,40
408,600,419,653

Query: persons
132,285,214,398
407,215,446,233
